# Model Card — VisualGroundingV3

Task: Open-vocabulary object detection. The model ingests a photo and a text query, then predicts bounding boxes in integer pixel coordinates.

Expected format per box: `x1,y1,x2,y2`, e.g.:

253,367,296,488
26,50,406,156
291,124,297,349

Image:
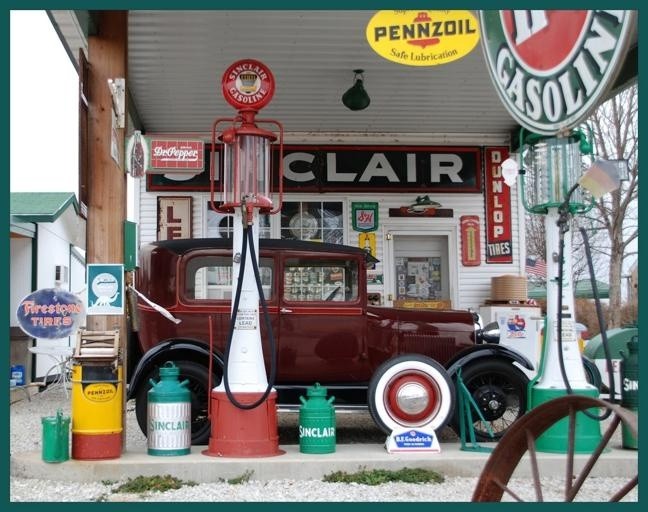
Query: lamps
341,69,370,112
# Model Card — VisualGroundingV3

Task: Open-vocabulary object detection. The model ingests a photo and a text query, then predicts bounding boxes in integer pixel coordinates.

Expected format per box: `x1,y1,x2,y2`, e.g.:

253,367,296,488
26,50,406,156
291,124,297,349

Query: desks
27,346,74,398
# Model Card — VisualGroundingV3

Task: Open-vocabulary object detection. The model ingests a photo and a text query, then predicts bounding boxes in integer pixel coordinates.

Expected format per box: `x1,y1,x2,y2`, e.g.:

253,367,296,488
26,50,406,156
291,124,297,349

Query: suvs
123,238,537,465
